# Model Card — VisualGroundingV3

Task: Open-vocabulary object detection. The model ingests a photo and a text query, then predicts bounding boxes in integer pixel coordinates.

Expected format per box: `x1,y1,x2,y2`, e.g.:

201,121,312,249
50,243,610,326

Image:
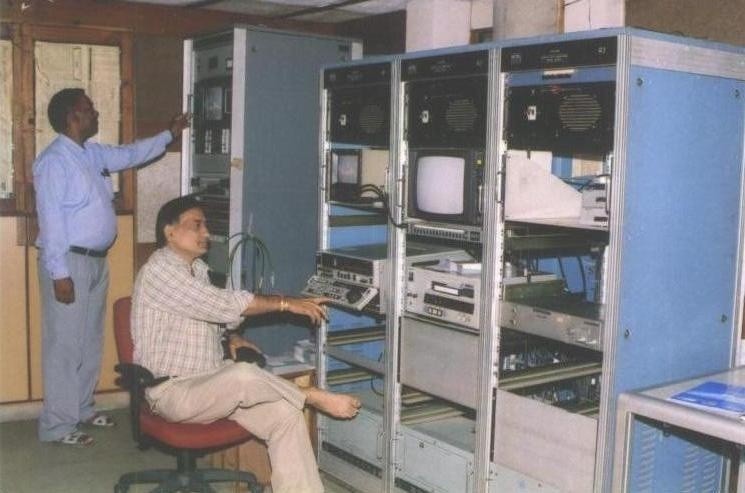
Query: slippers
81,413,114,427
55,431,94,444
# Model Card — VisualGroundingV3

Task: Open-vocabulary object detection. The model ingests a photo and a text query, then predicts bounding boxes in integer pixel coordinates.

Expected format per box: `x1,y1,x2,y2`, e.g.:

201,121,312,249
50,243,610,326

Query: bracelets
281,294,288,310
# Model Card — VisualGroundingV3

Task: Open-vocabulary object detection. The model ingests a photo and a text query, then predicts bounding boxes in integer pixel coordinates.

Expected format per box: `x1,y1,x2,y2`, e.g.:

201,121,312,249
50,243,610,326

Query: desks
614,365,745,493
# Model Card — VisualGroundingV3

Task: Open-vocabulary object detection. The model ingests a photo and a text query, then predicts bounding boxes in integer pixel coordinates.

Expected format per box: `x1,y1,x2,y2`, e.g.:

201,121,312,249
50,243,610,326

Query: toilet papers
504,149,581,221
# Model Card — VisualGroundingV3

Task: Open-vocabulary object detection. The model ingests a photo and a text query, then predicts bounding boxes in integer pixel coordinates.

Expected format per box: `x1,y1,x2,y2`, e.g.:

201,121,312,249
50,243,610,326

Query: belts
68,246,108,257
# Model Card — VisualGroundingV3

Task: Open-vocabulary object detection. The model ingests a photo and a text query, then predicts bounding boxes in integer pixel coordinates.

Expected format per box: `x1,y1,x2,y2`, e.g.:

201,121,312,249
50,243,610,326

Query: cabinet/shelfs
179,24,363,359
313,28,745,493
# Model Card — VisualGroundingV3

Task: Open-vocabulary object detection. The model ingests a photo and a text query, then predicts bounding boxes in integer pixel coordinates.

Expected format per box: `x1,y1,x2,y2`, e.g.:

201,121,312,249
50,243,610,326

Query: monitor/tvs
408,146,486,226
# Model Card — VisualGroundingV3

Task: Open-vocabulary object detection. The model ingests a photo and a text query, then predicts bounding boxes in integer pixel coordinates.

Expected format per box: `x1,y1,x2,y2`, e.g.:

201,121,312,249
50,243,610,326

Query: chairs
112,297,265,493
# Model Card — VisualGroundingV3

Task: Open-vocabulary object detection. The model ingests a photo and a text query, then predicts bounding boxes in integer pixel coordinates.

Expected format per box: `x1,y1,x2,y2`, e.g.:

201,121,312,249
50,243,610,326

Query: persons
131,196,361,493
32,89,192,446
